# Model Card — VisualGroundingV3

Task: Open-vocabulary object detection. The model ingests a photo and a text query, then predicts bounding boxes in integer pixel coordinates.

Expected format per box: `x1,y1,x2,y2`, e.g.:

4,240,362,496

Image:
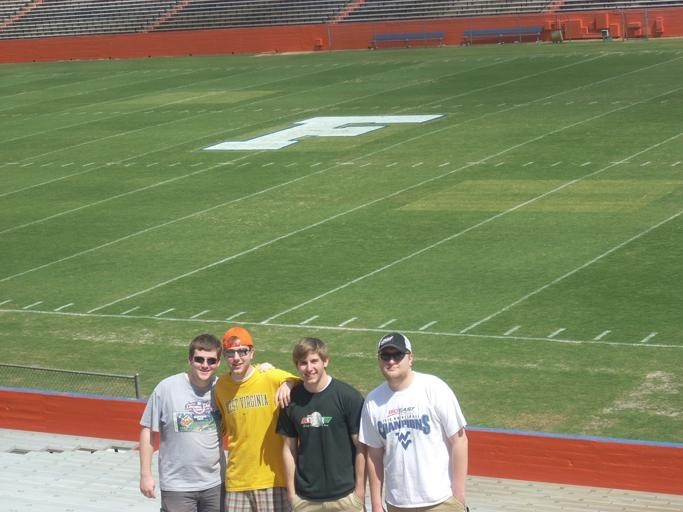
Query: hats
378,332,412,355
223,326,254,348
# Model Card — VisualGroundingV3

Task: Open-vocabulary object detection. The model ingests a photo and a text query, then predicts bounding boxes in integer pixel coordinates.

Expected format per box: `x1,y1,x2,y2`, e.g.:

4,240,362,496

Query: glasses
380,353,406,359
225,348,250,357
194,356,218,366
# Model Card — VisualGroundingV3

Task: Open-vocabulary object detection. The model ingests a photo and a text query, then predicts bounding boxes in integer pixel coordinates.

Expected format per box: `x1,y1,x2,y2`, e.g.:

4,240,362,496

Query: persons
139,333,276,512
274,336,370,512
357,331,470,511
213,325,305,512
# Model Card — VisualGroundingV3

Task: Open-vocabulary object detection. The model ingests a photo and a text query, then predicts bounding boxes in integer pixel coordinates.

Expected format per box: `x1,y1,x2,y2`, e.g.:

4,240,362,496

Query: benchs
368,31,444,50
463,27,543,46
